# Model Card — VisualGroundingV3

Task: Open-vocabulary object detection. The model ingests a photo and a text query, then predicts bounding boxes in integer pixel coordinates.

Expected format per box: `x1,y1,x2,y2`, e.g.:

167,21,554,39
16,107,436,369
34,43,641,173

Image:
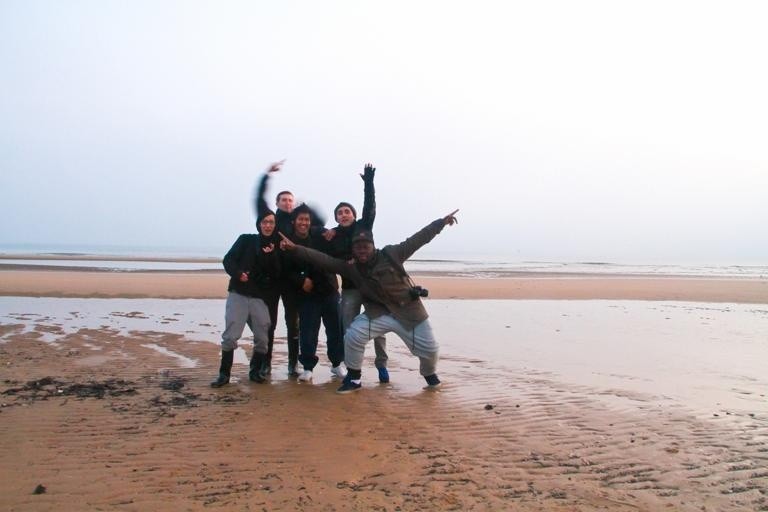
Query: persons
287,202,348,381
211,213,285,388
255,159,298,376
310,163,389,385
278,209,459,395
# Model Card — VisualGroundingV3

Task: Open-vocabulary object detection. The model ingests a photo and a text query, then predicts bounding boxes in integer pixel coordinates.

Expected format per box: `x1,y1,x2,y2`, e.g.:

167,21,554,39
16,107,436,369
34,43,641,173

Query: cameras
408,284,429,301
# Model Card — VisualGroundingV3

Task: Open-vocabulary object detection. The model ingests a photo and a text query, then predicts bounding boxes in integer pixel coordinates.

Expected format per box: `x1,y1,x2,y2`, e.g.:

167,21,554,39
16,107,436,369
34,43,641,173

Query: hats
351,231,374,243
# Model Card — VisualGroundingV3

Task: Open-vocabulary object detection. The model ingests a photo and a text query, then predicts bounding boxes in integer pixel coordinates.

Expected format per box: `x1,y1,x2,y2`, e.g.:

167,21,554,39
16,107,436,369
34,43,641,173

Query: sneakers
298,370,313,381
330,365,348,377
378,367,390,383
263,358,273,375
287,359,303,378
424,373,443,387
335,379,366,393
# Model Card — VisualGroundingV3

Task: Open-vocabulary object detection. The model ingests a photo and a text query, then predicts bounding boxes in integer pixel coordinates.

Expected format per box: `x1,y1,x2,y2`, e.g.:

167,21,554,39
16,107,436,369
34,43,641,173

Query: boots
249,351,268,385
211,350,234,389
